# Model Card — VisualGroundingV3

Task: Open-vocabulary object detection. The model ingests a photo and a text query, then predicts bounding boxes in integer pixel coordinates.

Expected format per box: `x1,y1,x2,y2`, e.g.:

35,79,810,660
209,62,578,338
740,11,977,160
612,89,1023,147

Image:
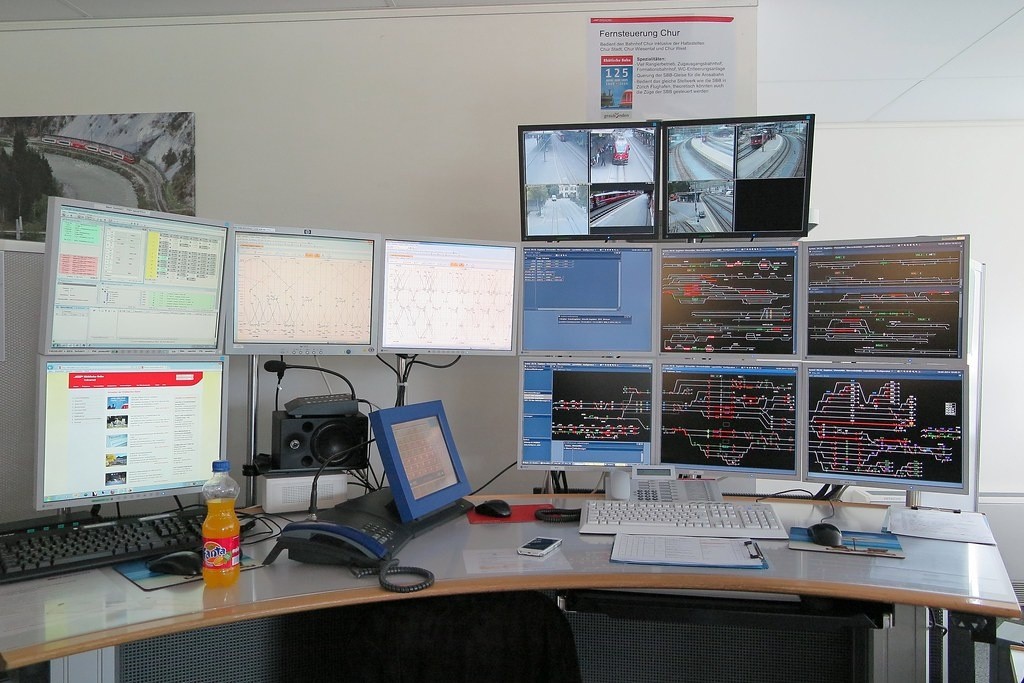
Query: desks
0,493,1024,683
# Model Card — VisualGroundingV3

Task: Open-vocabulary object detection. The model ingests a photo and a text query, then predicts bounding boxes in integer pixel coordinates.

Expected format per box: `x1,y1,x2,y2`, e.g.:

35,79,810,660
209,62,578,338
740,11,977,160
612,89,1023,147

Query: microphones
265,360,356,401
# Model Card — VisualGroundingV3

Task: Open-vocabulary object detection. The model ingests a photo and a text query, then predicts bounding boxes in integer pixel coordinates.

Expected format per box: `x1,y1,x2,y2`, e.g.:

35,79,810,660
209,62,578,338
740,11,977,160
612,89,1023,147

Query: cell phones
516,537,563,557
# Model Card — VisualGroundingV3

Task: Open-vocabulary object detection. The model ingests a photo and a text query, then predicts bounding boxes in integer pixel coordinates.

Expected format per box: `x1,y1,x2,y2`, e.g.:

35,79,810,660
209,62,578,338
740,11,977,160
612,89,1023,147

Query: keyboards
0,506,208,585
578,500,790,540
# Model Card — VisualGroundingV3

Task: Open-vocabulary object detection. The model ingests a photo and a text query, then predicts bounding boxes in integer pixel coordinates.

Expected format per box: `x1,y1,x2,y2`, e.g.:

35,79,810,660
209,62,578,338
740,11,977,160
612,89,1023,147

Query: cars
699,211,705,218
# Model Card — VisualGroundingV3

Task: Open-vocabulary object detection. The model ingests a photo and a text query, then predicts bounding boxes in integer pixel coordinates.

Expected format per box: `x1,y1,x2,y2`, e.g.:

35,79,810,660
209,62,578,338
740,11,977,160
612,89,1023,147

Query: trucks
669,193,677,201
694,133,702,138
552,194,557,201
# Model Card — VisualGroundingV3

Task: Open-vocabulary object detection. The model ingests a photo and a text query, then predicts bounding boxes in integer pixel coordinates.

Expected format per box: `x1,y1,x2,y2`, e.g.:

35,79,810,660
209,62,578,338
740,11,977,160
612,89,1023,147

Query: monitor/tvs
518,242,659,358
517,357,656,471
368,399,473,524
803,233,973,365
37,195,230,354
377,235,523,354
662,113,817,240
224,223,383,355
517,120,662,242
658,241,803,360
32,354,230,511
801,362,970,496
657,361,801,481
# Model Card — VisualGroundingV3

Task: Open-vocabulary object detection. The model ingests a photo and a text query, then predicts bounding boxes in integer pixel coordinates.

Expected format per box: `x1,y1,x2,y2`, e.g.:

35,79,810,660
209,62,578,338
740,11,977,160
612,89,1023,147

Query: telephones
605,466,680,501
276,508,436,592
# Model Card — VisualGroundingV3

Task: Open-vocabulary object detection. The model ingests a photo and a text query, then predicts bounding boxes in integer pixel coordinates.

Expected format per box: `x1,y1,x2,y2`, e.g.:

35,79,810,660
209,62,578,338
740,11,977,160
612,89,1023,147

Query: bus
669,134,682,143
41,136,134,164
750,122,798,149
612,137,629,165
590,190,637,213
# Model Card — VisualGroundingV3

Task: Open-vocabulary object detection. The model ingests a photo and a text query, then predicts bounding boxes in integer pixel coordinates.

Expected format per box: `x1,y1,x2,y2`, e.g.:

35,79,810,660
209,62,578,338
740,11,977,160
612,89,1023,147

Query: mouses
474,498,513,518
146,550,203,576
806,523,843,548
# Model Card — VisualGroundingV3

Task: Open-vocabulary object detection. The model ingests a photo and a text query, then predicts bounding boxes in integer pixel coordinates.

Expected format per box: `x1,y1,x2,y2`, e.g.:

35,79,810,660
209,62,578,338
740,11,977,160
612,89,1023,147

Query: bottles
202,460,240,589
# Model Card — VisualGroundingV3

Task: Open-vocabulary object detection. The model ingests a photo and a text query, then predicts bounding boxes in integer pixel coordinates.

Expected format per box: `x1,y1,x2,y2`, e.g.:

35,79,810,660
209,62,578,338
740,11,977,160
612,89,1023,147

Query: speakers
271,409,369,473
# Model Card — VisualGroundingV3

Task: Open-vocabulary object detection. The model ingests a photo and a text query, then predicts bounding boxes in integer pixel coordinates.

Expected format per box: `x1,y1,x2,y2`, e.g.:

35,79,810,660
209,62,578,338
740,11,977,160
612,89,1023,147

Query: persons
600,145,613,166
